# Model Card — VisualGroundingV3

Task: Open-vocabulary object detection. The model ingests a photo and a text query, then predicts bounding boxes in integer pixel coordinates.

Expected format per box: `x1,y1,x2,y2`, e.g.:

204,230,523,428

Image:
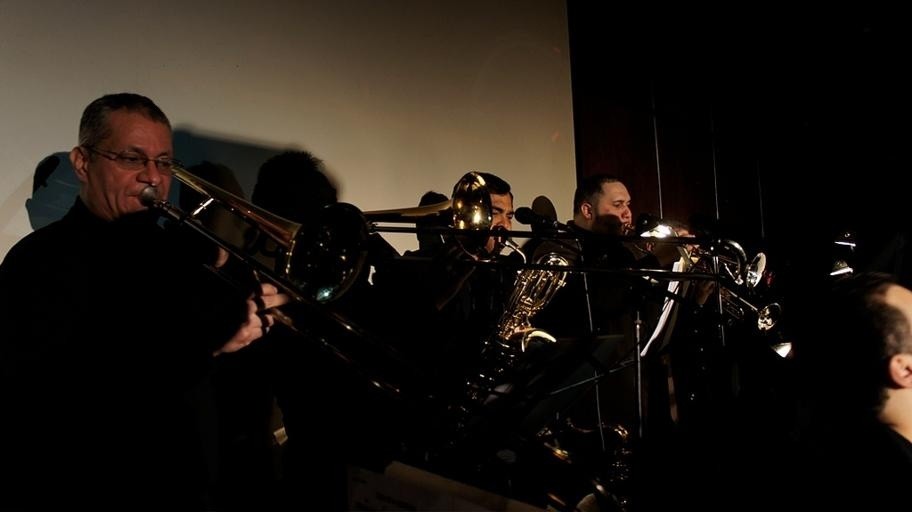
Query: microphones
514,206,575,234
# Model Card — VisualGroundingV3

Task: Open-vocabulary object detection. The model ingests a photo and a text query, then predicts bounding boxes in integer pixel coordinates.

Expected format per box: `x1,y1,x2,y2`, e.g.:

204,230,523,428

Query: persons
785,268,911,510
377,173,536,504
0,93,298,508
545,173,723,510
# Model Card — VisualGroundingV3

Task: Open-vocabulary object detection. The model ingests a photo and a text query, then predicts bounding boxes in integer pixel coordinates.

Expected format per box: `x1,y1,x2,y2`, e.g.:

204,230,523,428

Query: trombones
358,173,747,284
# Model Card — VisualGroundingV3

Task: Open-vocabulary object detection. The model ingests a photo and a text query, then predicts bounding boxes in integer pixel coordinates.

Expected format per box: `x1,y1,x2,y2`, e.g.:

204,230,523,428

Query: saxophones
458,239,570,419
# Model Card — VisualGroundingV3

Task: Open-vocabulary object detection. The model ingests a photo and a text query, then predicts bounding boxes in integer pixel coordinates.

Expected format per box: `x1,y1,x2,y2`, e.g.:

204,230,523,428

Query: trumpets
719,289,781,332
138,163,415,393
683,244,767,290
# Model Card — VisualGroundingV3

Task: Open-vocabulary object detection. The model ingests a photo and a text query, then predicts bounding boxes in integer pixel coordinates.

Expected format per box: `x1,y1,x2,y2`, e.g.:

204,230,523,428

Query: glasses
86,144,186,176
